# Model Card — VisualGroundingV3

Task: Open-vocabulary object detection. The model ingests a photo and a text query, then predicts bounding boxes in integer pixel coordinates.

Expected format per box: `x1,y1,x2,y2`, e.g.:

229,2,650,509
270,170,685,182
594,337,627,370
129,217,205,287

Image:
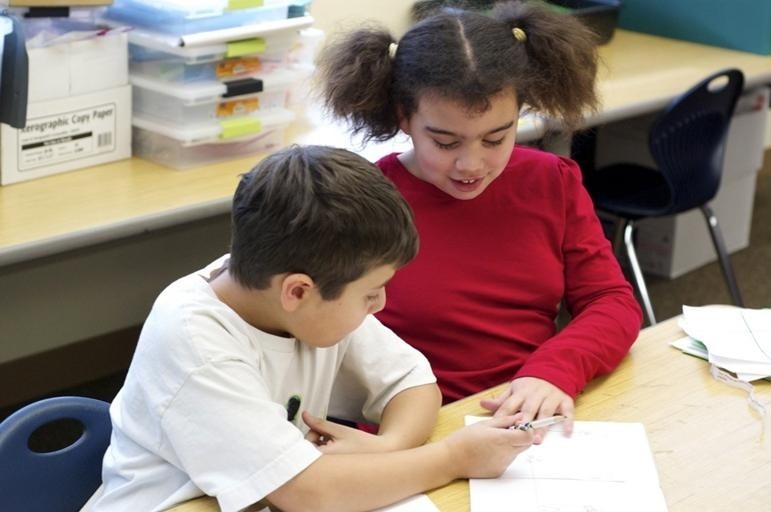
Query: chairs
2,395,108,512
582,67,746,326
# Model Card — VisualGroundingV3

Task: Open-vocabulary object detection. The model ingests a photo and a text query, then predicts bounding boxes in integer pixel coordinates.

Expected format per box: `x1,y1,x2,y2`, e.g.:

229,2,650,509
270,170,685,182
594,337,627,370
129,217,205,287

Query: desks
0,105,550,366
161,305,771,512
551,28,771,279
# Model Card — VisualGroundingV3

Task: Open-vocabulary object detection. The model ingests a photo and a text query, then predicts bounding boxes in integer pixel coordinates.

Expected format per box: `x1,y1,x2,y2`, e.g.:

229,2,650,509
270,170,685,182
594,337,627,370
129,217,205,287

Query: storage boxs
128,66,296,127
131,105,298,172
0,31,133,186
71,7,314,87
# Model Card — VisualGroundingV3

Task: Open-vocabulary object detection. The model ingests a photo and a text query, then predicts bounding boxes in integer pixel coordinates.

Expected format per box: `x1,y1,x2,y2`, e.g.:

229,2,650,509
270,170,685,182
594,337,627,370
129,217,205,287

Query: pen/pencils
504,414,568,432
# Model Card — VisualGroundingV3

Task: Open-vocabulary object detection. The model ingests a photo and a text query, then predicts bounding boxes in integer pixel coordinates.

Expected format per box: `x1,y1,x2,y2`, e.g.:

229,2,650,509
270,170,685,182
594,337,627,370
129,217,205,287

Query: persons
314,0,645,443
78,146,536,512
